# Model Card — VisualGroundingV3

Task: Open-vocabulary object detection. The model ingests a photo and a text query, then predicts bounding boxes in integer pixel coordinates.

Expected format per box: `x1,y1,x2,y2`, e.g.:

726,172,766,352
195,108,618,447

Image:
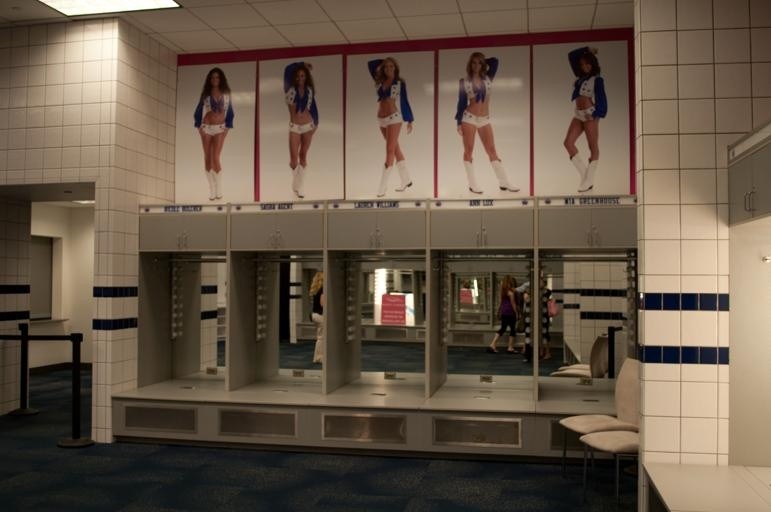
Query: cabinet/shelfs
721,117,771,226
135,209,230,252
324,207,428,252
427,206,536,252
106,374,616,470
536,204,639,249
229,207,325,252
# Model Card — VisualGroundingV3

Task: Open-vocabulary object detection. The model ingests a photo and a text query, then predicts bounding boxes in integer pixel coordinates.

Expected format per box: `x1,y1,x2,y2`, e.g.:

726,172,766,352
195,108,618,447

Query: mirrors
167,252,228,381
344,252,425,379
438,252,535,377
251,252,323,377
540,249,640,381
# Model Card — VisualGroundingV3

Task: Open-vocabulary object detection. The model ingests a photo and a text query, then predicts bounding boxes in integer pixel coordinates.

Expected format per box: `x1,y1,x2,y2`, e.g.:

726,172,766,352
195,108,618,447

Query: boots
464,159,484,194
215,168,224,199
570,152,599,193
205,168,216,201
289,162,308,198
395,159,413,192
491,159,520,192
377,162,393,197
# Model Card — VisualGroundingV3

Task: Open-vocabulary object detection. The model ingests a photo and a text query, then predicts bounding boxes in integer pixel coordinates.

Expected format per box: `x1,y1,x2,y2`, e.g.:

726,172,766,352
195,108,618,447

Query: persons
454,51,521,194
563,45,608,192
489,274,552,363
283,61,318,198
194,67,235,200
309,271,324,365
368,57,414,197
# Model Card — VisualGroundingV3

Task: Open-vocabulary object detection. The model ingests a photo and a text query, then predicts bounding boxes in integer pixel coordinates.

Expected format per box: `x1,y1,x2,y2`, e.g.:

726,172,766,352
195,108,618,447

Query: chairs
549,334,609,378
555,356,640,481
555,329,611,371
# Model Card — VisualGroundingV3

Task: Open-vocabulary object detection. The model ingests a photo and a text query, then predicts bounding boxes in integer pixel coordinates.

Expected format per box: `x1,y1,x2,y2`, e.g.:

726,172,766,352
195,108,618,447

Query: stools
576,424,642,511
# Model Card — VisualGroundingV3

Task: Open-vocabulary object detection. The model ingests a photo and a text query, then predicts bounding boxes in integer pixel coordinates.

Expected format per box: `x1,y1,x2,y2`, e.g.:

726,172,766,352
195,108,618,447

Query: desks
641,459,770,511
